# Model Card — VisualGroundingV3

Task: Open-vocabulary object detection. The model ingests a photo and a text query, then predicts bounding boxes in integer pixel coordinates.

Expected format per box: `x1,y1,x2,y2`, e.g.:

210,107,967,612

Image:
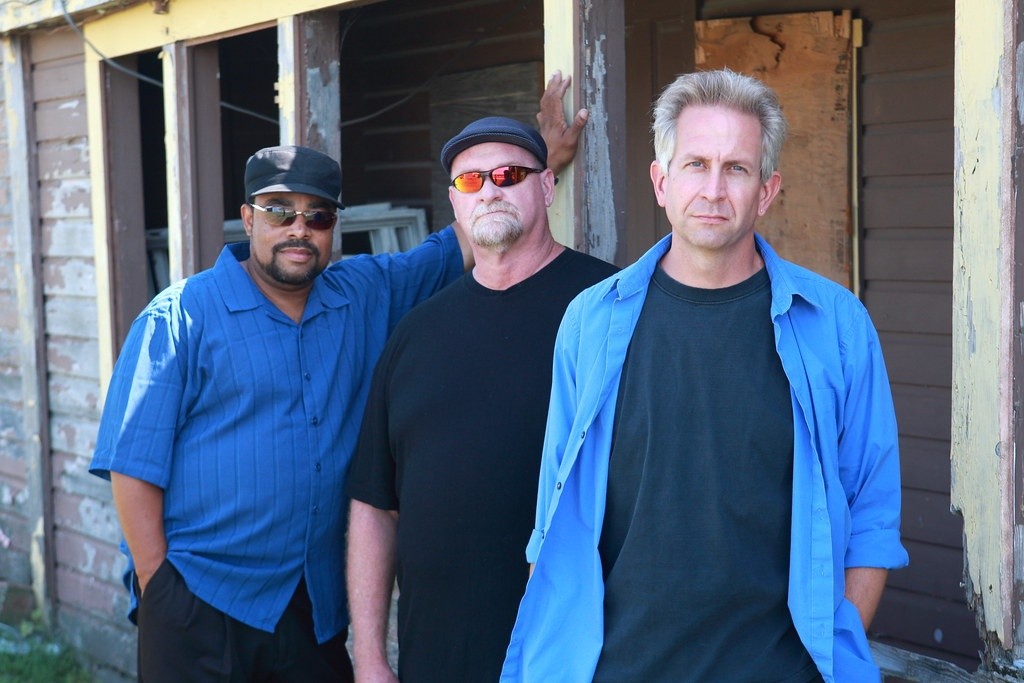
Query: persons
341,116,625,683
90,73,588,683
499,70,909,683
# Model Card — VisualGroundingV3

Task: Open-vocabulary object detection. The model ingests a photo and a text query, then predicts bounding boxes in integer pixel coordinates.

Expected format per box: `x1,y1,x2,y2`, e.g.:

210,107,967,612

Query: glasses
249,201,338,231
451,166,543,193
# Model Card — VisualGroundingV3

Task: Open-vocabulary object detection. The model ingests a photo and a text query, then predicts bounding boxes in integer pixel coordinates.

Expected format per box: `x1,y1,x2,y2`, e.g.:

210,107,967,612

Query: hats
244,145,345,211
441,116,548,181
